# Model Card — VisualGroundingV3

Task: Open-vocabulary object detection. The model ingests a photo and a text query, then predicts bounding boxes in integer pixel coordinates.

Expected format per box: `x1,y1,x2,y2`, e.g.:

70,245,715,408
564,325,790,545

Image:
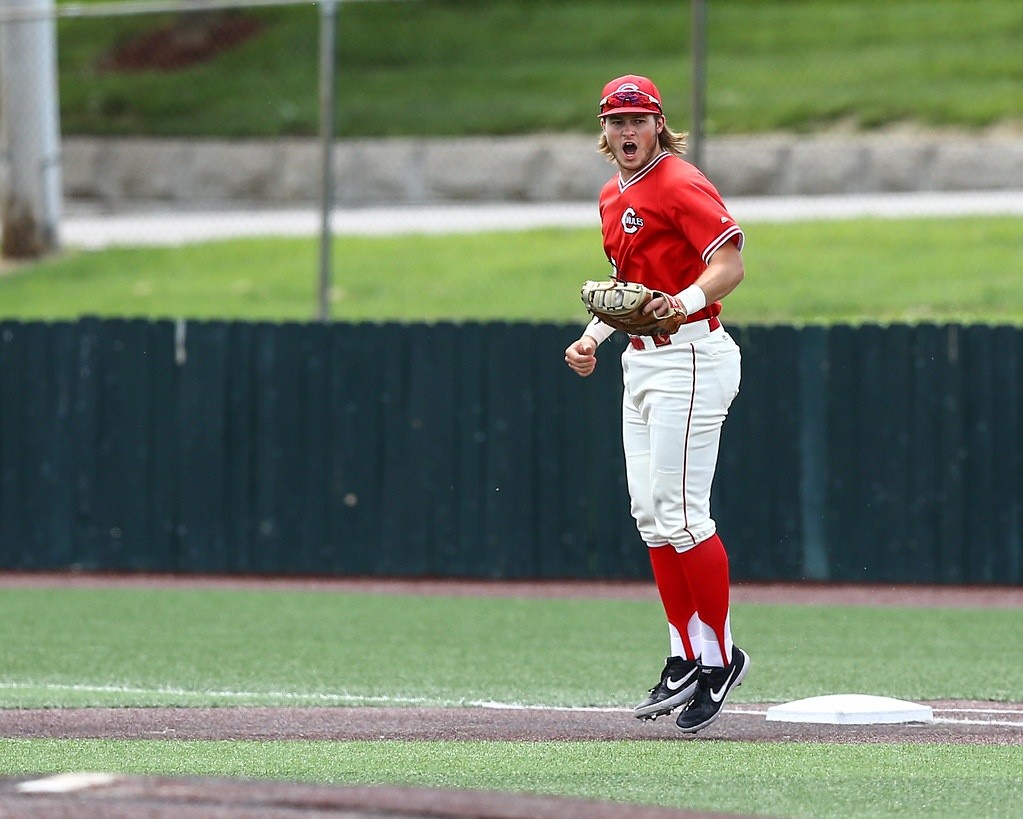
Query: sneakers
675,645,751,733
634,653,703,723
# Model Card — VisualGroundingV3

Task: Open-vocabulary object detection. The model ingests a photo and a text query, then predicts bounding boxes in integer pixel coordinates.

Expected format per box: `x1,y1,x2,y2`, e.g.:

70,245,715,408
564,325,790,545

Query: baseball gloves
581,260,688,337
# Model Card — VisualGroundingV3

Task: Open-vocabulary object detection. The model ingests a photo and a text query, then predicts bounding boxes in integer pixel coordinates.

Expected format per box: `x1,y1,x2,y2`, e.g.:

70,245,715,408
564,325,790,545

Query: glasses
600,89,663,115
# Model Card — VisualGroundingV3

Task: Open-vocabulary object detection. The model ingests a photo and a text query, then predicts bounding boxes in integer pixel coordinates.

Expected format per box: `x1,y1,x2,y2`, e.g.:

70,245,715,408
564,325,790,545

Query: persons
564,74,750,733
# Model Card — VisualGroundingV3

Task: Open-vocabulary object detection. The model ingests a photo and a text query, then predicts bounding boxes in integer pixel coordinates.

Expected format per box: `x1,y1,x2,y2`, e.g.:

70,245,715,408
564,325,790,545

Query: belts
630,316,718,350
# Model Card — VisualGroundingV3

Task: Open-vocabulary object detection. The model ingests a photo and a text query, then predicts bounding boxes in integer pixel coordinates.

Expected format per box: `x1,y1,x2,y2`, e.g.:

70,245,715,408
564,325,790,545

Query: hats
596,75,664,118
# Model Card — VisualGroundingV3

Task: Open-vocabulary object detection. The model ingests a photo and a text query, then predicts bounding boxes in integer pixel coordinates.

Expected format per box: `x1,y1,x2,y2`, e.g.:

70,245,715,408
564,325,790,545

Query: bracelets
674,283,707,317
582,309,618,348
